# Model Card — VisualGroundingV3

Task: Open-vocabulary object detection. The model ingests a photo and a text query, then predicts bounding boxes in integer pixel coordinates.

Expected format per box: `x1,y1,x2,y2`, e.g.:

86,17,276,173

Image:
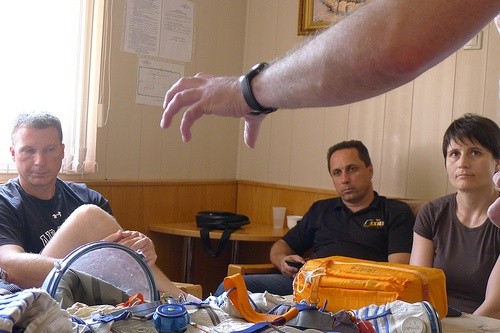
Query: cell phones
285,259,304,268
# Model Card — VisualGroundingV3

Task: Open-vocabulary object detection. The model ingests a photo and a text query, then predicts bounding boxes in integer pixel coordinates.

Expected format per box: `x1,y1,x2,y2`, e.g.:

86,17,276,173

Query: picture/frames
297,0,373,36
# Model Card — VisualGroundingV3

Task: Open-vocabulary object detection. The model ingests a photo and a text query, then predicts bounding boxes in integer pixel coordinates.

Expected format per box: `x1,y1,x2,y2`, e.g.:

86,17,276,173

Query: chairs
169,263,277,301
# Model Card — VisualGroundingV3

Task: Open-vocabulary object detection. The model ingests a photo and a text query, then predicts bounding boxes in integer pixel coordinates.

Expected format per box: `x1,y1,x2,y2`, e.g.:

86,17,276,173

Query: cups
273,207,286,228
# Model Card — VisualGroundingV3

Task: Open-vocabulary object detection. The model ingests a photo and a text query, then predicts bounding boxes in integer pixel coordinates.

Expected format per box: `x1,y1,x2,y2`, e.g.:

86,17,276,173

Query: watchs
239,62,278,116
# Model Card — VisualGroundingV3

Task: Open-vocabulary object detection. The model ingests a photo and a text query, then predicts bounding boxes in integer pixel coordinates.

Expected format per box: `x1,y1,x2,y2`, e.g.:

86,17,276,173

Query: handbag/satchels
196,211,251,258
292,255,449,319
348,300,442,333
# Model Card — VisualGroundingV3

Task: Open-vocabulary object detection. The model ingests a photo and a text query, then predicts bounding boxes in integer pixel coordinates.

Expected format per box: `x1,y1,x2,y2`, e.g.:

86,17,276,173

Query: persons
408,113,500,318
0,111,187,299
159,0,500,149
213,139,415,301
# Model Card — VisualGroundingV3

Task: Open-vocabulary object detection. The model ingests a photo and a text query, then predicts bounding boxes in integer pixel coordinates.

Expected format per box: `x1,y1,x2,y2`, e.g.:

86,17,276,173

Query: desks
149,222,291,283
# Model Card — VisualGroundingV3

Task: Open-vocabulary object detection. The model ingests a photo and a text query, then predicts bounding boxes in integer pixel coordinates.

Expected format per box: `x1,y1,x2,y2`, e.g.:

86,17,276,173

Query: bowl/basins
287,215,303,229
